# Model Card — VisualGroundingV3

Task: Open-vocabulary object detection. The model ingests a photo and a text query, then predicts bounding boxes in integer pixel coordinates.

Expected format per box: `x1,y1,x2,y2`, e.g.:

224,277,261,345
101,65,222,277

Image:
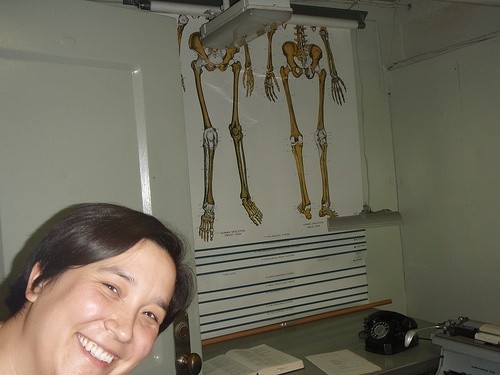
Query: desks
197,307,441,375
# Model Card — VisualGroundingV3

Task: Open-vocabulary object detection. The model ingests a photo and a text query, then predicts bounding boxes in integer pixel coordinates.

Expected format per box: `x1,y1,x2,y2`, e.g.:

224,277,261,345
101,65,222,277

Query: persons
0,202,199,375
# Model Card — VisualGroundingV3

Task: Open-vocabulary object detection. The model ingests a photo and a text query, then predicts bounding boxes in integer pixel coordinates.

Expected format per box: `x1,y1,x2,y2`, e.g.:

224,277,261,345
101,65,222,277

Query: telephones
361,309,419,357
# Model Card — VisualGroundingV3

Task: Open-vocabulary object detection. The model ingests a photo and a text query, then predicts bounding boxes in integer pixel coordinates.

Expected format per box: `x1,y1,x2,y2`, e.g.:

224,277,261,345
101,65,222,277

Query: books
200,342,304,375
